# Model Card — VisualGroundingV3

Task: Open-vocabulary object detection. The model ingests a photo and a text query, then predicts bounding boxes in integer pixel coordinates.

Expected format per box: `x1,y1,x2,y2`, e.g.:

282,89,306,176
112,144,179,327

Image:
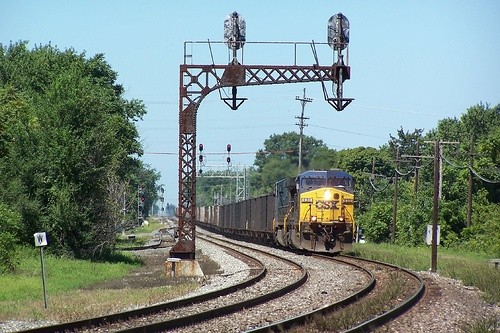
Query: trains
174,168,355,258
